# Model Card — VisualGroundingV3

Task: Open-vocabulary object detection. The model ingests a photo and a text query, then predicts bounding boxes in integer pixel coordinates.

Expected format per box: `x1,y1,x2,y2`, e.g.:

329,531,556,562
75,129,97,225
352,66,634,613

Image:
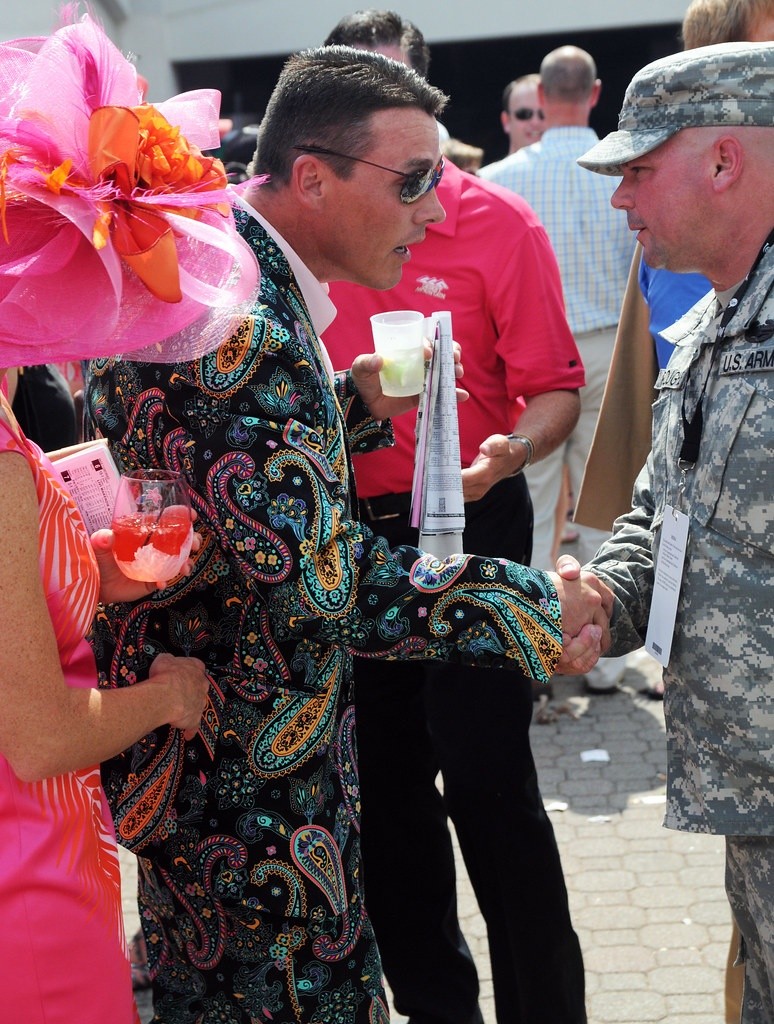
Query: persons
0,17,214,1021
80,46,598,1024
476,0,774,1024
245,11,587,1024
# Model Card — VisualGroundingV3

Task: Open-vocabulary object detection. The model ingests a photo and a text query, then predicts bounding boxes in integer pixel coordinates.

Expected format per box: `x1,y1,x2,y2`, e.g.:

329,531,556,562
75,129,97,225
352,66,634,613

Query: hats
0,14,270,364
576,41,774,176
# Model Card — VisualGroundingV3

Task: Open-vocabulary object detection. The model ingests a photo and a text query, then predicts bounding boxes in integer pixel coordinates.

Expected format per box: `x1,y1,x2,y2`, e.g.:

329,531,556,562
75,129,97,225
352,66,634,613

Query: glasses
512,107,545,121
294,145,446,204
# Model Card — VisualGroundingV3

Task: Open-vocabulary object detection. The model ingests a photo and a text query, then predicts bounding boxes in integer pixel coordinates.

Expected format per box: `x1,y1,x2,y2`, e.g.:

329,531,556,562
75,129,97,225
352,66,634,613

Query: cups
112,470,193,583
370,310,425,396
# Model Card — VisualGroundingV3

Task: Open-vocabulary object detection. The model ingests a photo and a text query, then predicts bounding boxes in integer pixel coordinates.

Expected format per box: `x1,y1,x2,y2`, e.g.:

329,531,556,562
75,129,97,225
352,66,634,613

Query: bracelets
505,430,536,478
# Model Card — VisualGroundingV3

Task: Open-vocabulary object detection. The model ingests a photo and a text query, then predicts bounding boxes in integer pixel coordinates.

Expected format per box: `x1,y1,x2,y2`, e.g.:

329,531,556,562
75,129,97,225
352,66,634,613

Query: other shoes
583,678,625,694
532,683,554,702
